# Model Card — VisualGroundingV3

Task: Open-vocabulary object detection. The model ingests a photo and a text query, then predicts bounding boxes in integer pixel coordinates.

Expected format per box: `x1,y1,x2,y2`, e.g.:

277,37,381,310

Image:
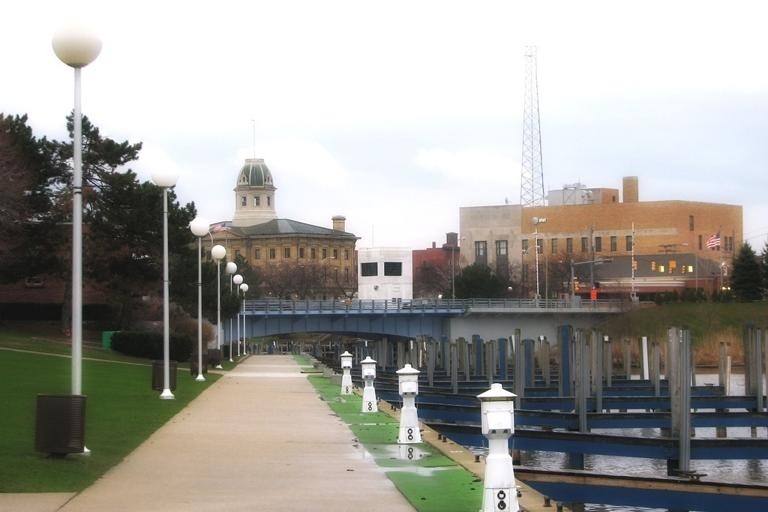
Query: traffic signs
591,287,598,299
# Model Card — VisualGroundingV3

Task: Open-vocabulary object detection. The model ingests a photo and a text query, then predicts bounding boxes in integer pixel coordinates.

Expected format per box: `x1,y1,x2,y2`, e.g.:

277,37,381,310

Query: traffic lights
575,281,580,290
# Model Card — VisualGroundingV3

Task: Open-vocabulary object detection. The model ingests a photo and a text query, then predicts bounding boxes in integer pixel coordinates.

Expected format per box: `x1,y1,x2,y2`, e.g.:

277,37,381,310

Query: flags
213,222,226,233
705,232,720,248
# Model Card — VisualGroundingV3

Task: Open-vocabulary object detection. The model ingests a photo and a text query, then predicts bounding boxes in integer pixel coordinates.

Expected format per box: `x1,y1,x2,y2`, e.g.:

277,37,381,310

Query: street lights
450,237,465,307
321,255,336,271
47,19,104,457
533,217,546,300
188,215,212,383
147,158,181,399
208,243,251,371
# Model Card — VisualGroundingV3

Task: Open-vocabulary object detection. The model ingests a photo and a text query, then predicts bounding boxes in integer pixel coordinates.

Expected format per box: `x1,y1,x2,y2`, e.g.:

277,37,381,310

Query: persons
590,286,598,308
